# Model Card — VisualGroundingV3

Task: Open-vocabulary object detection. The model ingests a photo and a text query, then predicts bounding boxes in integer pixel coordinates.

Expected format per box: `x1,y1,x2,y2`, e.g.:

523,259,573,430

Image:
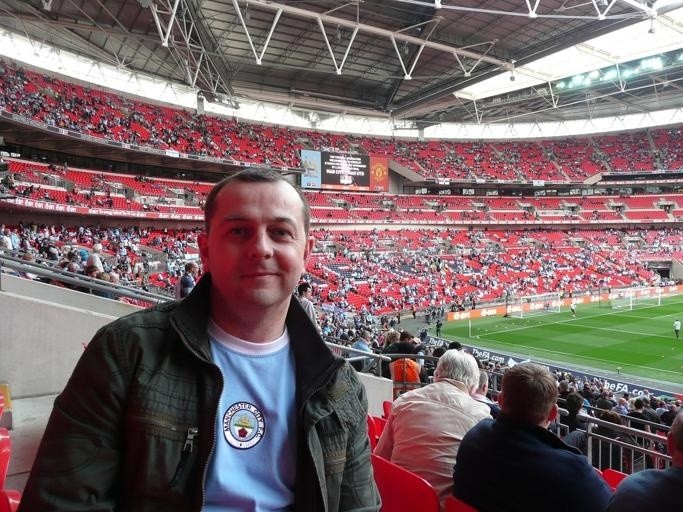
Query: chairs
363,414,629,507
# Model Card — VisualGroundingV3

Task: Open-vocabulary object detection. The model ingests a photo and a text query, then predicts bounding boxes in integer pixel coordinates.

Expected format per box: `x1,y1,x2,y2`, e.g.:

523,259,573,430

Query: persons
325,333,682,511
18,167,381,511
1,60,683,342
